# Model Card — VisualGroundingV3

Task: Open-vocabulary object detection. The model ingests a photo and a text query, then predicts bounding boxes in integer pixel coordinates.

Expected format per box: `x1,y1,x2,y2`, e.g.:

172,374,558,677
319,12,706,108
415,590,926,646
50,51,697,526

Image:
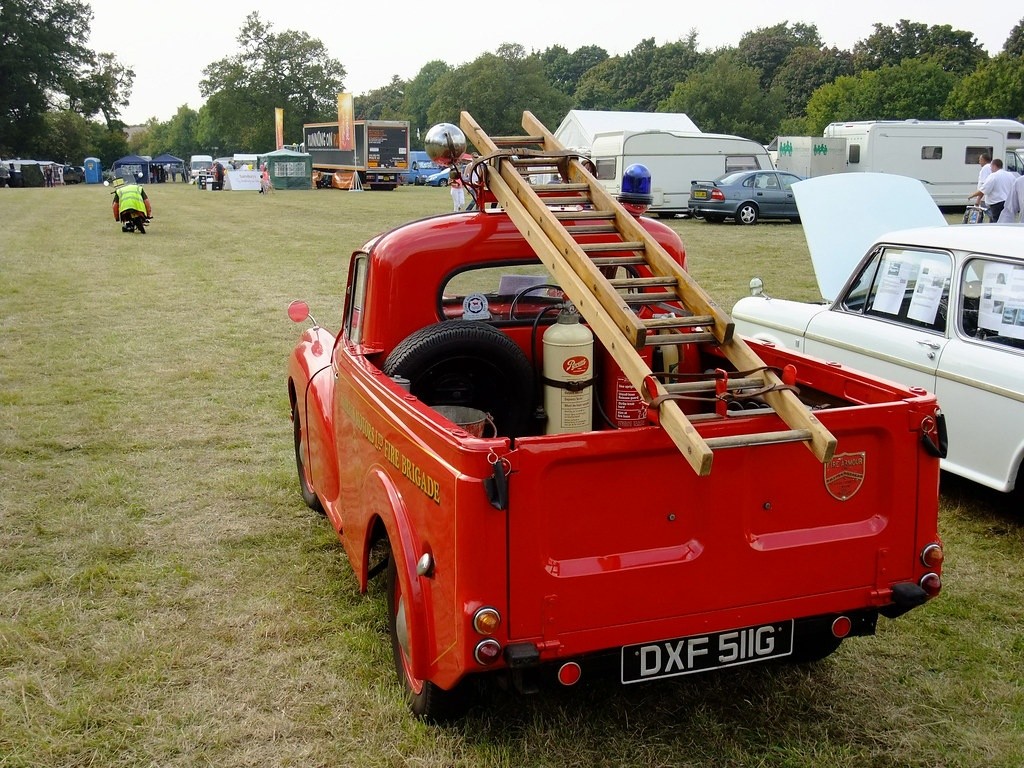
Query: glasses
473,156,479,158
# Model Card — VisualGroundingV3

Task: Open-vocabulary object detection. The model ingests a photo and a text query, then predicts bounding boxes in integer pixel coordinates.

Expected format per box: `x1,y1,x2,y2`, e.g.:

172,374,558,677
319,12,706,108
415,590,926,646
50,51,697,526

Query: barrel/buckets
428,405,497,441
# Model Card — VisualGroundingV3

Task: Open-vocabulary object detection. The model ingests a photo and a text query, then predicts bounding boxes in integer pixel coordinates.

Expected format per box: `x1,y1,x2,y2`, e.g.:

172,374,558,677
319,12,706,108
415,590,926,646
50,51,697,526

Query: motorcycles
103,168,154,234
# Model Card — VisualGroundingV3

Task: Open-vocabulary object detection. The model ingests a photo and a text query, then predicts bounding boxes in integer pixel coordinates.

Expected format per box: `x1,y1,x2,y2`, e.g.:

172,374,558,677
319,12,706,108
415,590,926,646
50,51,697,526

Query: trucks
823,118,1024,206
398,151,441,186
300,120,410,191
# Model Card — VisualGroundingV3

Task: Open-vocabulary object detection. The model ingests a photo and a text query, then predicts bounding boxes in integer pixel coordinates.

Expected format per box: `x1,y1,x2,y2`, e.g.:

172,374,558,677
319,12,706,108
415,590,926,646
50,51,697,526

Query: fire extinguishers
600,298,701,429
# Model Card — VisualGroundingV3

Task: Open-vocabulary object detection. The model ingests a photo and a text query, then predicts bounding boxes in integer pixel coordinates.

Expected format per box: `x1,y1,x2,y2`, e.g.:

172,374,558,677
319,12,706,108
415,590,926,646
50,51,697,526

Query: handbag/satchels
962,204,984,224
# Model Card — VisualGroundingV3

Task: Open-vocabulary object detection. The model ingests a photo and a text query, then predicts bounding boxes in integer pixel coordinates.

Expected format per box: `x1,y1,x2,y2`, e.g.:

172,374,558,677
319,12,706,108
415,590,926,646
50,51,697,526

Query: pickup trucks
63,166,86,184
286,163,950,729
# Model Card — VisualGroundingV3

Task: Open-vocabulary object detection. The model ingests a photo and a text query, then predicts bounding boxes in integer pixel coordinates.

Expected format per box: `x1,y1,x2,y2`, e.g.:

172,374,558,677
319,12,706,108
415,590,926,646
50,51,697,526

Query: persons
448,169,466,213
975,153,996,223
996,175,1024,225
969,159,1018,223
45,163,275,195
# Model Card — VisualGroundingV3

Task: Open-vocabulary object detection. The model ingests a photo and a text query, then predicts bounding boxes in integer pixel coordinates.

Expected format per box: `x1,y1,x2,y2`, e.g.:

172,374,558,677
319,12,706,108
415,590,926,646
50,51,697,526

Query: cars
688,170,804,225
425,165,467,187
730,171,1023,493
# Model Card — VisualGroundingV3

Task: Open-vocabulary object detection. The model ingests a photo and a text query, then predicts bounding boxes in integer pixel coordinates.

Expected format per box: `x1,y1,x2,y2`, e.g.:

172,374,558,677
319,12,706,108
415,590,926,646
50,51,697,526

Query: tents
256,148,312,190
112,154,149,184
149,154,186,183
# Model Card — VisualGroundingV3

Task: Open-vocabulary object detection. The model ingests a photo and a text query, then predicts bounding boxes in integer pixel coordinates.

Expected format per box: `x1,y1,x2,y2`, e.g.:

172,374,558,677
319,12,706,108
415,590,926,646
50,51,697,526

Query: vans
188,155,213,180
590,129,778,219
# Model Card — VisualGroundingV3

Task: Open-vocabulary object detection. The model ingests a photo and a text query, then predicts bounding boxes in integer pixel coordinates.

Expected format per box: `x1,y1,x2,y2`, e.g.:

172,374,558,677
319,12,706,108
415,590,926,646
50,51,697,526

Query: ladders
452,104,841,477
